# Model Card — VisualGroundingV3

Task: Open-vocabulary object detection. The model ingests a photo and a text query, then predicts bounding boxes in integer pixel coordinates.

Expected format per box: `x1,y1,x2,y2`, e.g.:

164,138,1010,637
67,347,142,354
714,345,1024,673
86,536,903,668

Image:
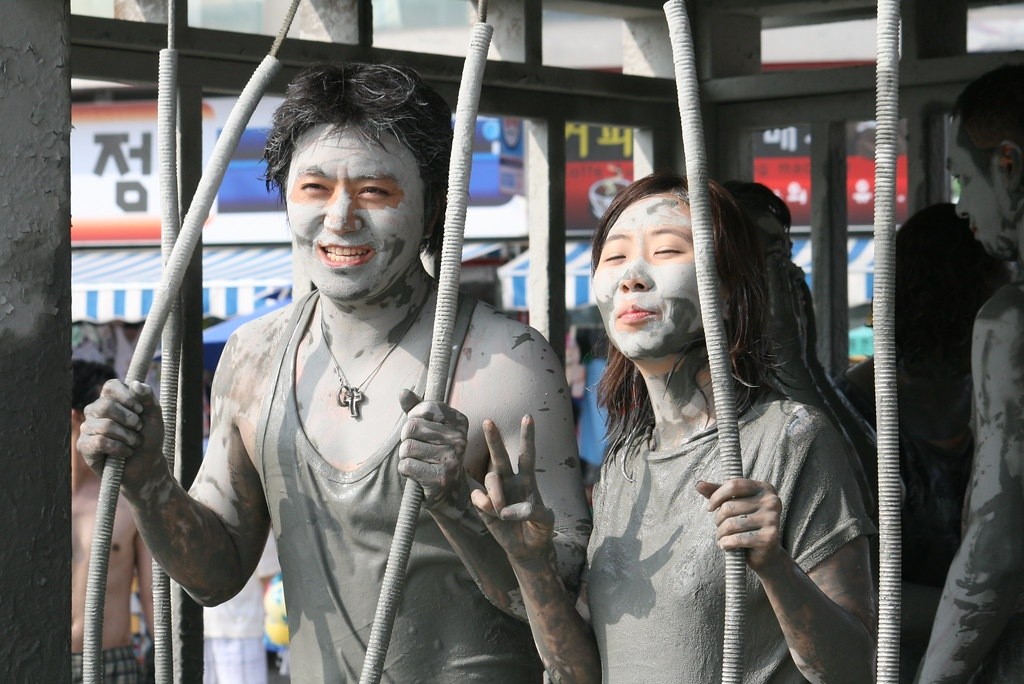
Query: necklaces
312,278,432,417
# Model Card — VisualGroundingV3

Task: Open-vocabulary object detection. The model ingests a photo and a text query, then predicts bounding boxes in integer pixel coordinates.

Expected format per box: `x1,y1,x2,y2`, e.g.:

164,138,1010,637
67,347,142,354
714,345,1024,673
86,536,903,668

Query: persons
70,360,158,684
720,59,1023,684
76,61,594,684
472,173,879,684
